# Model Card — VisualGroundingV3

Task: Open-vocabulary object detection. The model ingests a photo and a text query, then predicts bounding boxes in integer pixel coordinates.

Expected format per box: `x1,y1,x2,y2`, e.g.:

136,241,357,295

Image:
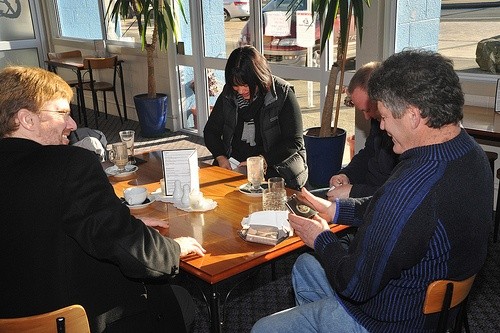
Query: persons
203,45,309,191
307,61,398,200
0,65,207,333
249,48,494,333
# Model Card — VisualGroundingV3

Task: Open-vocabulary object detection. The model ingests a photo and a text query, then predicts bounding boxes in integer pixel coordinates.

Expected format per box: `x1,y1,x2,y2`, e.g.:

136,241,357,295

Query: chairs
1,305,90,333
76,49,123,131
422,272,480,332
47,49,96,126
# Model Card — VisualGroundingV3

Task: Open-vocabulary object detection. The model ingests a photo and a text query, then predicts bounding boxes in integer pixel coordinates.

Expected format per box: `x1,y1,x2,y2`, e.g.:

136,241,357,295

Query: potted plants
103,0,188,133
282,0,373,187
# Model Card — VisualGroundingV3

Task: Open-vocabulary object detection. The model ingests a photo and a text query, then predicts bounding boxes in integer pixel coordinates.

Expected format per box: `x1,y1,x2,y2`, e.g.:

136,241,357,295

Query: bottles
180,184,191,206
172,181,183,201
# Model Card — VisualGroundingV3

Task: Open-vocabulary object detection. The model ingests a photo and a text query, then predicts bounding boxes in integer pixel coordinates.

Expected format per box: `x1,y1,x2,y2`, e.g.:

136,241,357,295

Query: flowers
331,54,356,108
188,66,219,99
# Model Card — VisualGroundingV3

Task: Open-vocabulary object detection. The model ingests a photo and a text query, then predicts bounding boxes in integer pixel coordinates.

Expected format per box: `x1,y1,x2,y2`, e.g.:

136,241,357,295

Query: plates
126,196,156,210
239,181,269,198
105,164,139,179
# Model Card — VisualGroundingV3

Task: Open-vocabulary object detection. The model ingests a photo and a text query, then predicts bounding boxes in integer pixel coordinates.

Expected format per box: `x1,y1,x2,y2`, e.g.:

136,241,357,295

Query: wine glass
108,142,128,173
246,156,264,191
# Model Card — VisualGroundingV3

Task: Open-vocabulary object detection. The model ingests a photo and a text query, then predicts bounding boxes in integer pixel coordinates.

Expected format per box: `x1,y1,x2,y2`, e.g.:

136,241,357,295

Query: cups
188,191,204,210
160,179,164,193
267,177,284,191
262,188,287,211
118,130,136,164
123,187,148,205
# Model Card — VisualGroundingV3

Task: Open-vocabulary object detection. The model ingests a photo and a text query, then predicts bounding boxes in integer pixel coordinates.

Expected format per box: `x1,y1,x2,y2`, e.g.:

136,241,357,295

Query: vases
189,105,214,128
347,135,357,159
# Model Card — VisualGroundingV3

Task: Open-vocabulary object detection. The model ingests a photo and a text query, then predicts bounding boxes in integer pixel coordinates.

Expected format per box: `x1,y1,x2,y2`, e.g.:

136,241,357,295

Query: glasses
36,109,71,120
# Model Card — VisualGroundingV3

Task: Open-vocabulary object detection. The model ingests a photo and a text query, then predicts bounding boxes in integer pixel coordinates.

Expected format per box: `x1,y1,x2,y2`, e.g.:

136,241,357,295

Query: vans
238,0,356,67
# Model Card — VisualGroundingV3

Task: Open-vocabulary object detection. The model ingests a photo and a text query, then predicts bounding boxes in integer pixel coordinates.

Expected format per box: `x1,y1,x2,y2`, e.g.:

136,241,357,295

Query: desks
460,105,500,222
96,147,354,332
45,57,126,130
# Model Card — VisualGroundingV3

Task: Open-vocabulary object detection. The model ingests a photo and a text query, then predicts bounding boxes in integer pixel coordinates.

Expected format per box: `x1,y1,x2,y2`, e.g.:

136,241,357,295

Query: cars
223,0,249,21
